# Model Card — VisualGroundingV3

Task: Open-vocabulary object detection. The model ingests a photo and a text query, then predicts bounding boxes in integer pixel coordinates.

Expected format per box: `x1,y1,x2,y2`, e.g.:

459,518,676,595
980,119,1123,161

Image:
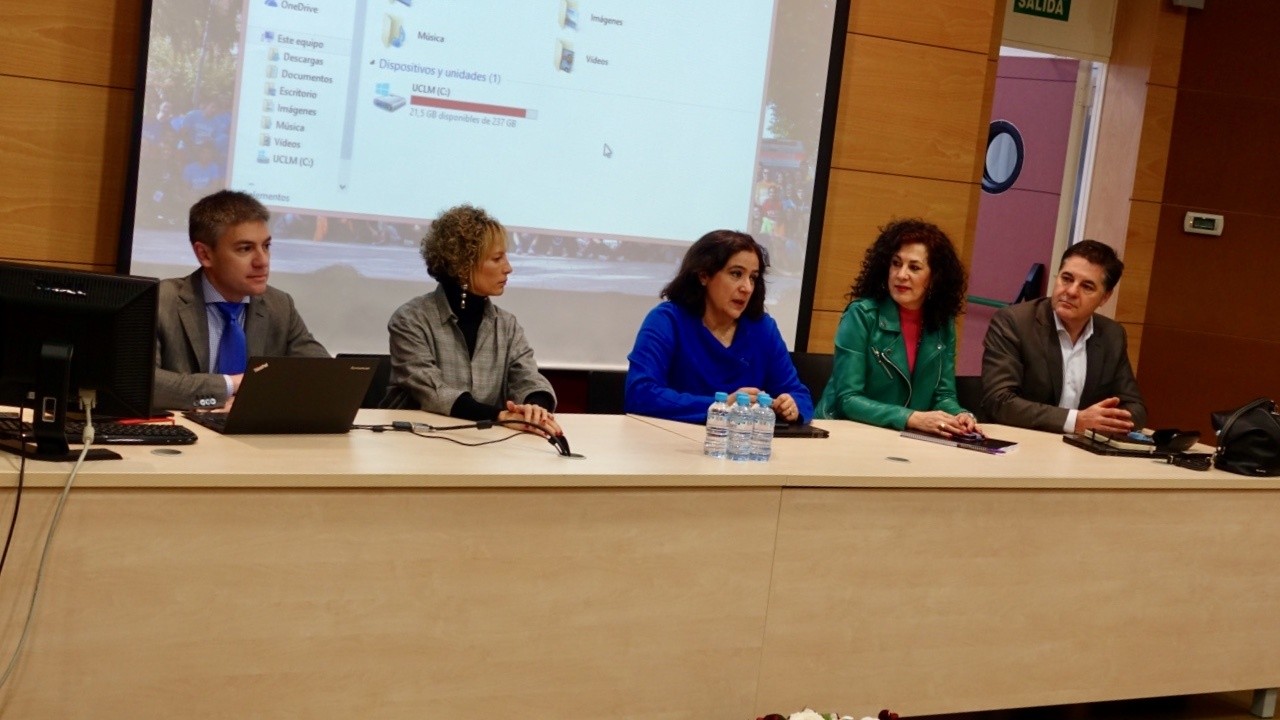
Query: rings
967,413,974,419
788,408,792,415
941,423,944,429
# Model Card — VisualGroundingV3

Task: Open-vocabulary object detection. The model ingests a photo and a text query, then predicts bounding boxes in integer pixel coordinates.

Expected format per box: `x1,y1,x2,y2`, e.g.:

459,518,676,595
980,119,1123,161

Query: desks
0,411,1280,720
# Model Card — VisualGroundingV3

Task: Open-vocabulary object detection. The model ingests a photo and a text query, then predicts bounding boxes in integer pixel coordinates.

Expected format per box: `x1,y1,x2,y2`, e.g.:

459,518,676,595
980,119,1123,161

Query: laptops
181,357,381,435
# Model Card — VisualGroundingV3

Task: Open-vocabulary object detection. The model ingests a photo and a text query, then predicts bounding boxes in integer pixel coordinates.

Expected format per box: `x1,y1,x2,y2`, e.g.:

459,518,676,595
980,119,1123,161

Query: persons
814,216,984,439
153,189,335,413
622,229,813,426
978,238,1147,434
386,203,565,438
141,94,810,263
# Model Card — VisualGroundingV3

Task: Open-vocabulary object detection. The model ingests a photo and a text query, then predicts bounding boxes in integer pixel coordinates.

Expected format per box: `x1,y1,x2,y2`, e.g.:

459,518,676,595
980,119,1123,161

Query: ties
211,304,248,374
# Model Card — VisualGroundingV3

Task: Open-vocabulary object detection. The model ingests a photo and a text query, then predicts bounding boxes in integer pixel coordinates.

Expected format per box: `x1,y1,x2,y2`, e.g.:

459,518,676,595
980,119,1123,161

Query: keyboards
0,420,198,445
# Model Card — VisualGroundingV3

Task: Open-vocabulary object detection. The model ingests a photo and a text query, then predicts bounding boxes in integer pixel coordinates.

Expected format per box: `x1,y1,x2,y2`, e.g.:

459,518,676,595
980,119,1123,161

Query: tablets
772,417,830,439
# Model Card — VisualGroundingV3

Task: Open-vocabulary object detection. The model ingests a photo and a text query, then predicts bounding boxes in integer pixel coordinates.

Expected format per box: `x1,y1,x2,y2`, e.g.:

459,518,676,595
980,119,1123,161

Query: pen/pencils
957,444,1006,453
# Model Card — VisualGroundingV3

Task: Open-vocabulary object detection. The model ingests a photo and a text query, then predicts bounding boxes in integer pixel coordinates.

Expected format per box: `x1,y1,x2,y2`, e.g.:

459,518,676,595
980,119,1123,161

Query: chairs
335,353,391,409
955,375,996,423
789,352,834,408
587,372,626,414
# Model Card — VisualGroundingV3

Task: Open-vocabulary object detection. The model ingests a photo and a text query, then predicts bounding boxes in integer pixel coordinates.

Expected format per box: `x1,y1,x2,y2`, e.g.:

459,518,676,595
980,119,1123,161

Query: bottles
703,391,776,463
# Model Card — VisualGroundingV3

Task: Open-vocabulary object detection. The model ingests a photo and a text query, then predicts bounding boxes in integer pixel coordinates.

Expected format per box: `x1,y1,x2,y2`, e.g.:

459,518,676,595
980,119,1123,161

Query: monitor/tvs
0,261,160,462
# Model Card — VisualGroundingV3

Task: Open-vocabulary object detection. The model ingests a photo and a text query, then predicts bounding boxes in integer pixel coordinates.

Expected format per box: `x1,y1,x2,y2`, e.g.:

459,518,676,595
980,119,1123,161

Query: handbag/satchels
1209,396,1280,477
